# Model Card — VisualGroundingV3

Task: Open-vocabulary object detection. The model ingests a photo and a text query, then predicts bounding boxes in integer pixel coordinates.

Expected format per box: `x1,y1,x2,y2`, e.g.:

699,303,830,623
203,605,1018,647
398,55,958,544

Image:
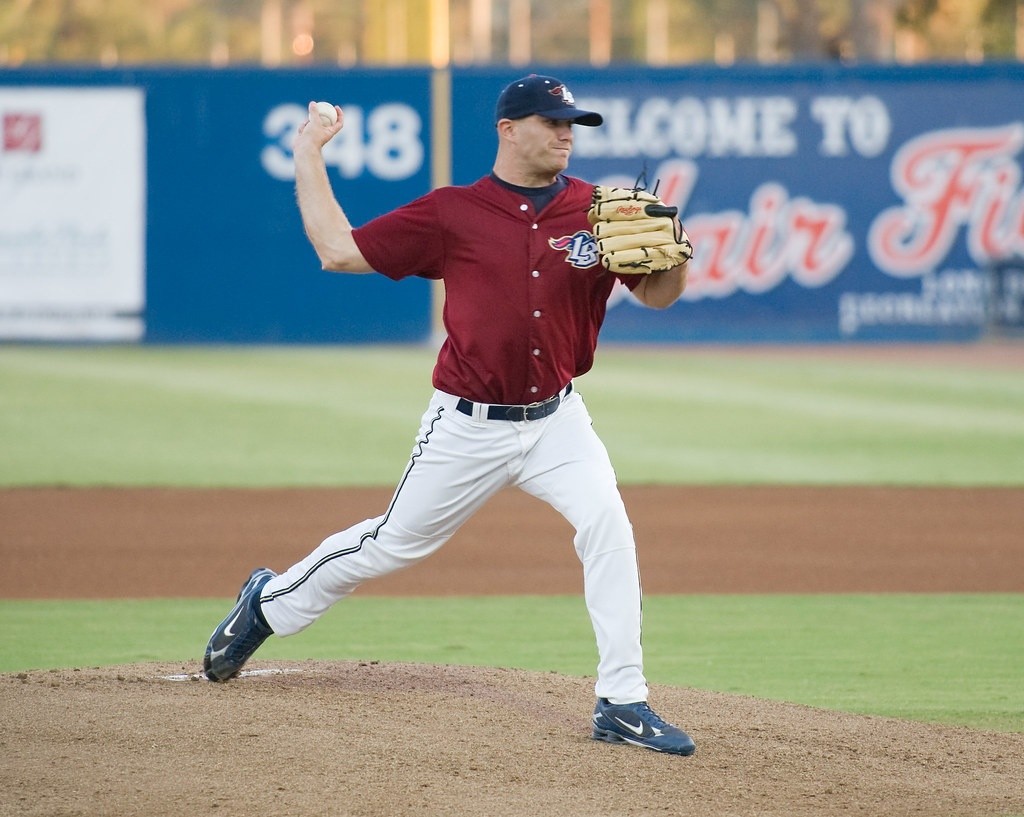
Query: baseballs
309,101,338,127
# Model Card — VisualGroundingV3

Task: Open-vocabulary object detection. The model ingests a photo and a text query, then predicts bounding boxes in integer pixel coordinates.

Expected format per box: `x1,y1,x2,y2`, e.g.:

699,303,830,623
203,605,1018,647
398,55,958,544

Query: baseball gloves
586,184,694,275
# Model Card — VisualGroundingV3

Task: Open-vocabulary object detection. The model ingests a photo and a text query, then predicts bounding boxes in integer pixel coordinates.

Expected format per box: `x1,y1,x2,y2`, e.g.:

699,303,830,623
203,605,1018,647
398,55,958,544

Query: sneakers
591,697,696,756
203,567,278,683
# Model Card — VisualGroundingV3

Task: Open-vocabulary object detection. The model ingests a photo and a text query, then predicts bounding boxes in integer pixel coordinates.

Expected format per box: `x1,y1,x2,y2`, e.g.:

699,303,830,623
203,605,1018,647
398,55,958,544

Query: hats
495,74,603,127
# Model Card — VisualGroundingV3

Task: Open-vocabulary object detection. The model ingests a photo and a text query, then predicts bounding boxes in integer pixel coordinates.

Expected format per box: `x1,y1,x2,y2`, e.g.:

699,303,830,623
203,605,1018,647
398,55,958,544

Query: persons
204,75,696,757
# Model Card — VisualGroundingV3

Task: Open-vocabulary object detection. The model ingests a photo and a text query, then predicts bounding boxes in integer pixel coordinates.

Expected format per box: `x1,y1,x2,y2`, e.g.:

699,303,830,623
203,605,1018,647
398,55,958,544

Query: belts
456,382,572,422
